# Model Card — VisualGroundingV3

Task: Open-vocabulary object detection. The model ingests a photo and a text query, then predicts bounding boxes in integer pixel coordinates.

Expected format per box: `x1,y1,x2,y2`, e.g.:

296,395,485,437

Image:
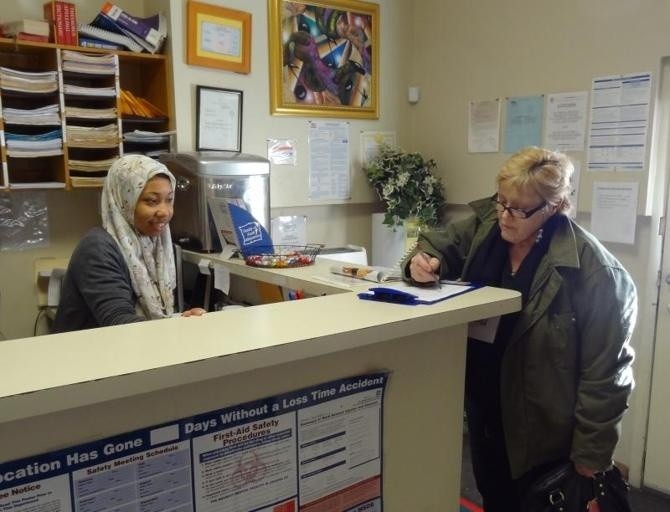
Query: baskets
232,243,323,269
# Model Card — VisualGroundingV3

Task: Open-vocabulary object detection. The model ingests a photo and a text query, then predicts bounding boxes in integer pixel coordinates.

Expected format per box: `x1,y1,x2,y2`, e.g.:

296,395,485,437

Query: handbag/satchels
520,454,631,511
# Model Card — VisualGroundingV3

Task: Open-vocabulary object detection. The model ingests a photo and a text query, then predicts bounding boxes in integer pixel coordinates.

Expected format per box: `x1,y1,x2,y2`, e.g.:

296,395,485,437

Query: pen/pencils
419,251,442,290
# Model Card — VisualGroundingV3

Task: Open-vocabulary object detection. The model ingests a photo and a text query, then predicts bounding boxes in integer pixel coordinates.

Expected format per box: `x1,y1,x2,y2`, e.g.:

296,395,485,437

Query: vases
372,206,403,268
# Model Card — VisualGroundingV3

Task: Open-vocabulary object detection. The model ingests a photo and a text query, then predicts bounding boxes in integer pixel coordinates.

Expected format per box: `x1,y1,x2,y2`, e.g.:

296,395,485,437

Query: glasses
490,192,547,220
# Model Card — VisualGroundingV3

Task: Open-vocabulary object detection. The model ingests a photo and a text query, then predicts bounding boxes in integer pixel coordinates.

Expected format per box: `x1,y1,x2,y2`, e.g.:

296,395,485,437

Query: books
0,1,173,189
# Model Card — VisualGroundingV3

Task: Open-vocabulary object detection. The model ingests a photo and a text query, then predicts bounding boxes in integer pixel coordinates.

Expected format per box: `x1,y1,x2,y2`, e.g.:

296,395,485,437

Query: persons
401,146,637,511
54,155,207,331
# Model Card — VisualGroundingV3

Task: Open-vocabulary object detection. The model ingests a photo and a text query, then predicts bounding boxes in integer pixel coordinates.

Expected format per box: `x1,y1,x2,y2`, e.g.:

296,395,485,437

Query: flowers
368,144,445,223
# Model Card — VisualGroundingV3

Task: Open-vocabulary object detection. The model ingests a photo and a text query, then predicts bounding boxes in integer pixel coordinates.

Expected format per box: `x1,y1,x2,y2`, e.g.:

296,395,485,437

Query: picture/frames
186,2,255,77
265,2,382,121
193,85,244,154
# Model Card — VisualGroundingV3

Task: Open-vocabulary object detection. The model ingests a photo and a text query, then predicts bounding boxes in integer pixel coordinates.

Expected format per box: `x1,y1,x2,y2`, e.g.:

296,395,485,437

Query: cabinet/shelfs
2,37,175,195
